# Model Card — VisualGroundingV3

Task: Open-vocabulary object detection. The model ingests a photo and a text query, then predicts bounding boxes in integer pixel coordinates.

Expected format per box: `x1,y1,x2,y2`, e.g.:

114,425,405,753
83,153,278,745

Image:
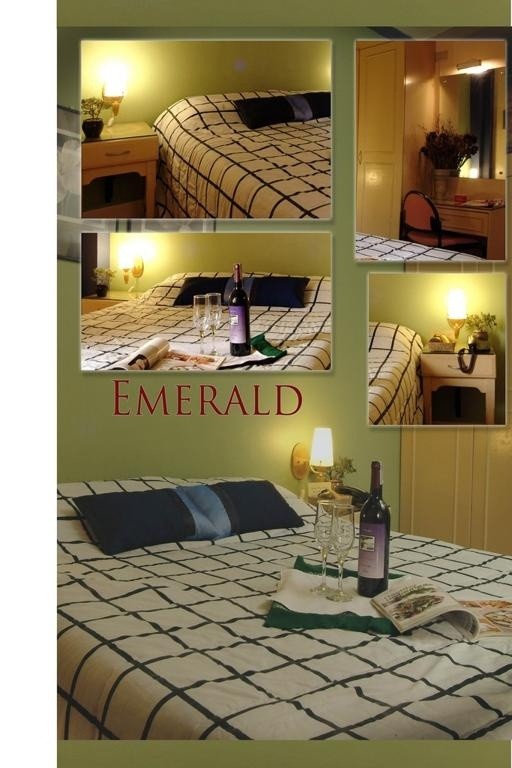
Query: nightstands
420,345,496,425
339,504,391,524
81,291,145,315
82,120,160,219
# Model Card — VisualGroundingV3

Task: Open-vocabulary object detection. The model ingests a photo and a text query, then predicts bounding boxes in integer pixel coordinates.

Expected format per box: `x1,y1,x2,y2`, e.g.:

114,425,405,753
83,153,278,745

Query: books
369,574,512,645
96,337,227,371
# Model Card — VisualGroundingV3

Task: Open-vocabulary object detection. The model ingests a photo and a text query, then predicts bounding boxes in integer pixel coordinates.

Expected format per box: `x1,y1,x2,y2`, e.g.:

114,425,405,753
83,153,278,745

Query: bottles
226,263,253,358
356,460,393,599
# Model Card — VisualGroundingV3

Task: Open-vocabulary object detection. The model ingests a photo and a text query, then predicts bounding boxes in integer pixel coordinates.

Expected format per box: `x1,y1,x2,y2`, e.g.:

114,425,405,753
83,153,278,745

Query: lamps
102,58,129,118
291,426,333,479
456,59,483,71
444,287,468,340
115,243,144,284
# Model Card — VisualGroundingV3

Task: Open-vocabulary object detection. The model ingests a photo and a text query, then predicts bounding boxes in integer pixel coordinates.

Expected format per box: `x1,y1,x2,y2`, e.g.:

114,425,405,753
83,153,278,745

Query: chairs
399,190,480,250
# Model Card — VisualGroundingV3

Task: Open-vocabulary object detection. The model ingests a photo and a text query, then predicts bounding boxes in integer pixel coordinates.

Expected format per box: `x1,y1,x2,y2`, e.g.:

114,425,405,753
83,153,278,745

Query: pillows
72,480,305,556
234,91,331,128
172,277,315,306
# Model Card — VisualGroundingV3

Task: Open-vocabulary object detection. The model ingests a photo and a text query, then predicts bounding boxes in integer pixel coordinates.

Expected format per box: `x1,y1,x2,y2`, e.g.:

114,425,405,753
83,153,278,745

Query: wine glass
191,292,222,358
311,498,354,603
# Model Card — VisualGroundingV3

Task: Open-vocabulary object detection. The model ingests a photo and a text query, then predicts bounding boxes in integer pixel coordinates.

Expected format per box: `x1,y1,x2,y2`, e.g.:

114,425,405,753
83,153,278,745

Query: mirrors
440,66,506,180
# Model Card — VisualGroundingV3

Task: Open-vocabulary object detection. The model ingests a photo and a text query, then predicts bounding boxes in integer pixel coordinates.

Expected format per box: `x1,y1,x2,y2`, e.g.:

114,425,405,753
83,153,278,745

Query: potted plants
92,267,115,297
325,455,356,487
81,97,111,139
464,310,497,353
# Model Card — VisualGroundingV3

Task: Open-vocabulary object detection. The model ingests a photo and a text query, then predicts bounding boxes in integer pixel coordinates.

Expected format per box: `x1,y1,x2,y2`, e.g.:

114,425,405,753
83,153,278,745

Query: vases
432,169,461,200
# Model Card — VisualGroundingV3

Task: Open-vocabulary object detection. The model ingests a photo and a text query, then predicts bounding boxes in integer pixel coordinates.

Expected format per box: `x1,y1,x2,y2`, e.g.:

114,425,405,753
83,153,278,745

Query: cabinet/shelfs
356,40,436,240
434,199,505,260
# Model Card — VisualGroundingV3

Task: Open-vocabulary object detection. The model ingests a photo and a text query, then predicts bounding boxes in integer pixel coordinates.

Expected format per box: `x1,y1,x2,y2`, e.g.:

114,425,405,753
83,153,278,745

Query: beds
81,271,331,372
152,89,331,218
57,477,511,739
369,322,424,425
356,232,486,260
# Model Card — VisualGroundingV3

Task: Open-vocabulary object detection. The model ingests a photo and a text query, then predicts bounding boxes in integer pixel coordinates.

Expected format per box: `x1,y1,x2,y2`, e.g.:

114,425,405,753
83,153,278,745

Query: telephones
335,486,370,507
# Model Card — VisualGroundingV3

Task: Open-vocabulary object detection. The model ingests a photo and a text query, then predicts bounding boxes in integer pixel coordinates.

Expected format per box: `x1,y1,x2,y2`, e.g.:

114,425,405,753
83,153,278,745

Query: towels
262,556,413,635
218,332,288,369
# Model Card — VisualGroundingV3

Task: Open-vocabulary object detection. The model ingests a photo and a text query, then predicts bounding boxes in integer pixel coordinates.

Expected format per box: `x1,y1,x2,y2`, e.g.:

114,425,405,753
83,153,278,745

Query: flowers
414,119,478,169
